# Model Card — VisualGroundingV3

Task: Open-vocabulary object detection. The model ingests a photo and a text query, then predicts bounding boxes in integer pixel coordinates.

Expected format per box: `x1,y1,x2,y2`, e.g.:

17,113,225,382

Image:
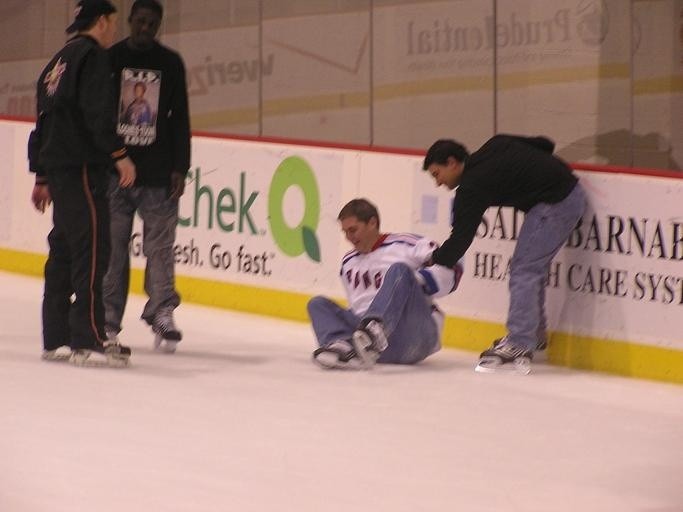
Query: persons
422,133,589,364
553,127,681,173
103,1,191,343
27,1,140,355
307,197,464,371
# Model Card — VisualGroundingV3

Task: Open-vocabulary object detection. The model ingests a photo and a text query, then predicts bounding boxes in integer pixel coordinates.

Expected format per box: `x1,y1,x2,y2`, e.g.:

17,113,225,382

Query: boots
146,298,183,343
477,332,549,369
40,337,133,357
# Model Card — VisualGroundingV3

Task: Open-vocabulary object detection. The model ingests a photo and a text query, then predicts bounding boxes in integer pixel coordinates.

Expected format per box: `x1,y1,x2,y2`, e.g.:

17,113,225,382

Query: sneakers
312,320,386,372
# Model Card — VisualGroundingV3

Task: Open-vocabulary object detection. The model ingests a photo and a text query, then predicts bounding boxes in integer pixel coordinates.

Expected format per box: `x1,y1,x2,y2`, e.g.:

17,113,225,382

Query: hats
64,0,118,34
